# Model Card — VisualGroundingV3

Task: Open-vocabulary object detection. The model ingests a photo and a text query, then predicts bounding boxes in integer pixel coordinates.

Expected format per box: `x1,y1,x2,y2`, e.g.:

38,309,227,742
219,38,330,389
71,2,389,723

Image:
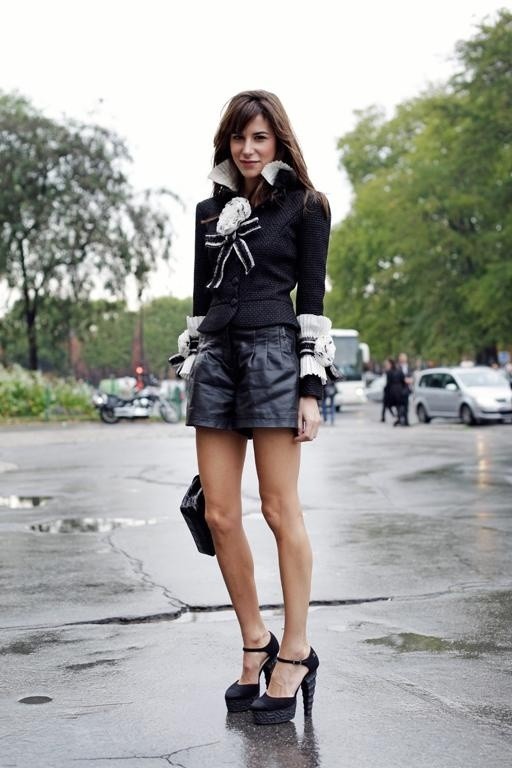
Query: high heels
224,631,278,713
248,645,319,727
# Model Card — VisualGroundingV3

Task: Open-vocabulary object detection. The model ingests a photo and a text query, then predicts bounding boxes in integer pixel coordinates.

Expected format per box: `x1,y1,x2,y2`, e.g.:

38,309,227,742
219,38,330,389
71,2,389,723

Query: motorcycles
91,365,181,423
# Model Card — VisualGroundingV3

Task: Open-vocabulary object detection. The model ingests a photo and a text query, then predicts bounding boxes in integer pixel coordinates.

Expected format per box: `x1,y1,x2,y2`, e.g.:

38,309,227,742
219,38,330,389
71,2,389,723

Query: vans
413,363,512,423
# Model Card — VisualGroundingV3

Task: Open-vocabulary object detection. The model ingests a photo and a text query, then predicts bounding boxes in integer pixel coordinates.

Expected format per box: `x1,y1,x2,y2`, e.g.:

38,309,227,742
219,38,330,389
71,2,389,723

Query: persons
167,88,332,726
320,353,512,427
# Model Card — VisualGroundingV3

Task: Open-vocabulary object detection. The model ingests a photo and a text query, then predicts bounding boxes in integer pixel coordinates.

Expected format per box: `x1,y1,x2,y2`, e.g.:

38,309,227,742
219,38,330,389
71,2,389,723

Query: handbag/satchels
181,478,217,555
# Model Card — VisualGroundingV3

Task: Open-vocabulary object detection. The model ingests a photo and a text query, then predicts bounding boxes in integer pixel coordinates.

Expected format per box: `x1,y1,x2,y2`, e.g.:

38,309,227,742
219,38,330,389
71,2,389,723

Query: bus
322,327,372,410
